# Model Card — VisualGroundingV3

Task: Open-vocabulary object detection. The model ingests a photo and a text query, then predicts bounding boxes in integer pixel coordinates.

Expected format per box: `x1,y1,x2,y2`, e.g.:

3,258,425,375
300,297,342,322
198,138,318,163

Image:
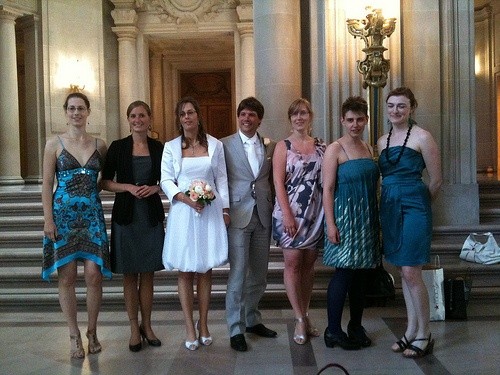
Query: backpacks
363,264,396,308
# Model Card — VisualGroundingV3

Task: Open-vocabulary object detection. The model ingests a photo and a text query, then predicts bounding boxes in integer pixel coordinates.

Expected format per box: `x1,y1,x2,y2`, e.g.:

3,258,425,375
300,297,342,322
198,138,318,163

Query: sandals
304,312,320,337
293,316,307,344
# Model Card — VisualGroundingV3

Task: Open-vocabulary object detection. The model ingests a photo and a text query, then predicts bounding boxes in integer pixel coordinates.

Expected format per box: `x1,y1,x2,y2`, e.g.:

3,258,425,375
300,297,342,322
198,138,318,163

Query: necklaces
386,124,412,165
188,141,200,154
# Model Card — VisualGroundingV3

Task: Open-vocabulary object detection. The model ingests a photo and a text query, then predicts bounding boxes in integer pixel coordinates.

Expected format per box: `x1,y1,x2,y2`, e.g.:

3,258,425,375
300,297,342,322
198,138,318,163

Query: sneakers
324,327,360,351
347,320,371,347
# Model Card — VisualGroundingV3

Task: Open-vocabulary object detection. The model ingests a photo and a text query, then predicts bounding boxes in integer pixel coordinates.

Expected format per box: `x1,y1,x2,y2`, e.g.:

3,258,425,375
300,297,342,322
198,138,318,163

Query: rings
286,229,288,231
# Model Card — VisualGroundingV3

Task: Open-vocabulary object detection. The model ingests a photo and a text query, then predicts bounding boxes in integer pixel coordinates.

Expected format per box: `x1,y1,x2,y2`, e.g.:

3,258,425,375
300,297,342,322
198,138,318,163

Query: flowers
183,177,217,218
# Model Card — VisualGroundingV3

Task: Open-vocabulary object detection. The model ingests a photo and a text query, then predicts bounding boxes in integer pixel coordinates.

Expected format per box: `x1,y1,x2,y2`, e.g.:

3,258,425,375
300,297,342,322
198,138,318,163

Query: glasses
179,110,196,118
292,111,309,116
67,106,86,113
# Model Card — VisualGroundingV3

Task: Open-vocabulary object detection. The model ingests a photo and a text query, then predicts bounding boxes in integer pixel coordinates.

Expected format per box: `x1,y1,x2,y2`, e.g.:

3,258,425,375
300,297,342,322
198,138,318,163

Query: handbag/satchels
458,231,500,265
445,278,467,322
421,254,446,322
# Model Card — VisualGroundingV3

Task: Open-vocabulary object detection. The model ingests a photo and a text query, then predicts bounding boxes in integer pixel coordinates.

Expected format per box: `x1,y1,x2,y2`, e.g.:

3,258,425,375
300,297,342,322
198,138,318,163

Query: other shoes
245,323,277,338
230,333,248,352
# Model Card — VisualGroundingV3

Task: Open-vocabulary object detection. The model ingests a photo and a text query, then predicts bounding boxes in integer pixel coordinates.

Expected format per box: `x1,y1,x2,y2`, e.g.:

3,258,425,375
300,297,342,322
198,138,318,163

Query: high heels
86,328,101,354
391,333,413,353
195,319,212,346
69,331,85,359
185,338,199,351
129,332,142,352
139,324,162,347
402,331,436,358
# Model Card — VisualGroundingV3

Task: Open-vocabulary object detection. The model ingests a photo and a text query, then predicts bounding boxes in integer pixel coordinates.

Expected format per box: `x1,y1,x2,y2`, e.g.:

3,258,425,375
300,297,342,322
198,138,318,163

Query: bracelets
223,212,230,217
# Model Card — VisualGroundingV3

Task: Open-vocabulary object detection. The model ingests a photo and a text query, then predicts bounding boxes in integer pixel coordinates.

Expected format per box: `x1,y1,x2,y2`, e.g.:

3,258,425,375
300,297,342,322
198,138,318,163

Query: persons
323,96,379,350
41,93,113,358
376,87,444,358
100,101,165,352
160,96,230,351
272,98,327,344
219,96,277,351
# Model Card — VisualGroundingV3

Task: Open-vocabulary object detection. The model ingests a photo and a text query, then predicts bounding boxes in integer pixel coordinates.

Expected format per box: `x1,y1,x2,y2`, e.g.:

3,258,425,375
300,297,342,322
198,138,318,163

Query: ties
245,138,260,179
